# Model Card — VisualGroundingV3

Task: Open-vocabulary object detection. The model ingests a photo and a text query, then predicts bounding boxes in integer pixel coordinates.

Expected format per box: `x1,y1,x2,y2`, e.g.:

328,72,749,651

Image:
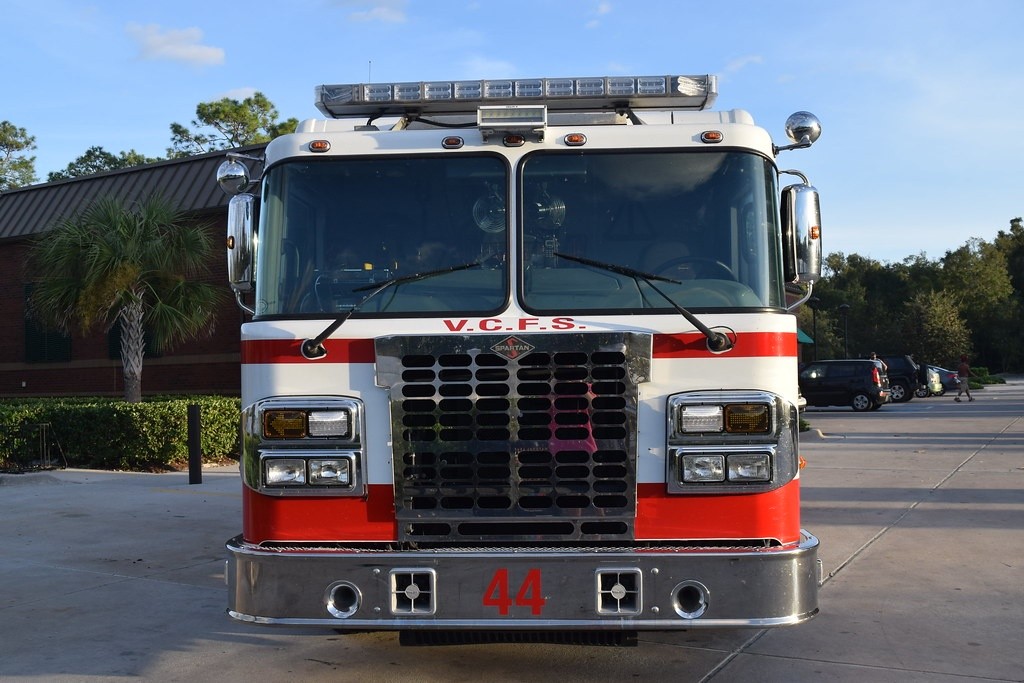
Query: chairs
597,240,696,279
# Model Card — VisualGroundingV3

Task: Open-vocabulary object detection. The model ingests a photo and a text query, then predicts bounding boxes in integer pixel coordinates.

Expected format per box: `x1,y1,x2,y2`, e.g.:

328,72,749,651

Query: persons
954,357,977,402
870,352,888,372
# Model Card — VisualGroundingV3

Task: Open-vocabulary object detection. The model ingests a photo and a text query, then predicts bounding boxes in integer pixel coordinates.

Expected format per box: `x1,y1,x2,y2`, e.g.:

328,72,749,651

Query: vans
798,360,891,413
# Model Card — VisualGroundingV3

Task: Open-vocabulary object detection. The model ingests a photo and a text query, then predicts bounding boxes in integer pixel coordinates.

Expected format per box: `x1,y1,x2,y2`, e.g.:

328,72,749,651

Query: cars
914,367,942,398
928,365,960,396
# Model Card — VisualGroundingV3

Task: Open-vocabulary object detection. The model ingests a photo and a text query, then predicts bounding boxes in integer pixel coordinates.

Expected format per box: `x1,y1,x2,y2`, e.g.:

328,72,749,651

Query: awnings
797,328,814,343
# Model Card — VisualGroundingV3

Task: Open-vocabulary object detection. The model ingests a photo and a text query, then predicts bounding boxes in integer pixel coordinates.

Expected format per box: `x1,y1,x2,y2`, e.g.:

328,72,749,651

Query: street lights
839,303,850,360
809,296,820,359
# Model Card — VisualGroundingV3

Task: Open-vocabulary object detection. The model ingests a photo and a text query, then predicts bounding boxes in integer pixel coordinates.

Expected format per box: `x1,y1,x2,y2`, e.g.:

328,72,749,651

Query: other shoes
954,398,961,402
969,397,975,401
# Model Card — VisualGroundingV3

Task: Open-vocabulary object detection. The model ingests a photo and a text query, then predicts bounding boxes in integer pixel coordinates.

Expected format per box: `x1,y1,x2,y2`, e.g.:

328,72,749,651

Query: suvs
861,355,929,403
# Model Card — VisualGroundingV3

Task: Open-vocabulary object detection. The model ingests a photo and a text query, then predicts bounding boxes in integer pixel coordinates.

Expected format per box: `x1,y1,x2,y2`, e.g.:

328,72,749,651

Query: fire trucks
215,73,823,629
